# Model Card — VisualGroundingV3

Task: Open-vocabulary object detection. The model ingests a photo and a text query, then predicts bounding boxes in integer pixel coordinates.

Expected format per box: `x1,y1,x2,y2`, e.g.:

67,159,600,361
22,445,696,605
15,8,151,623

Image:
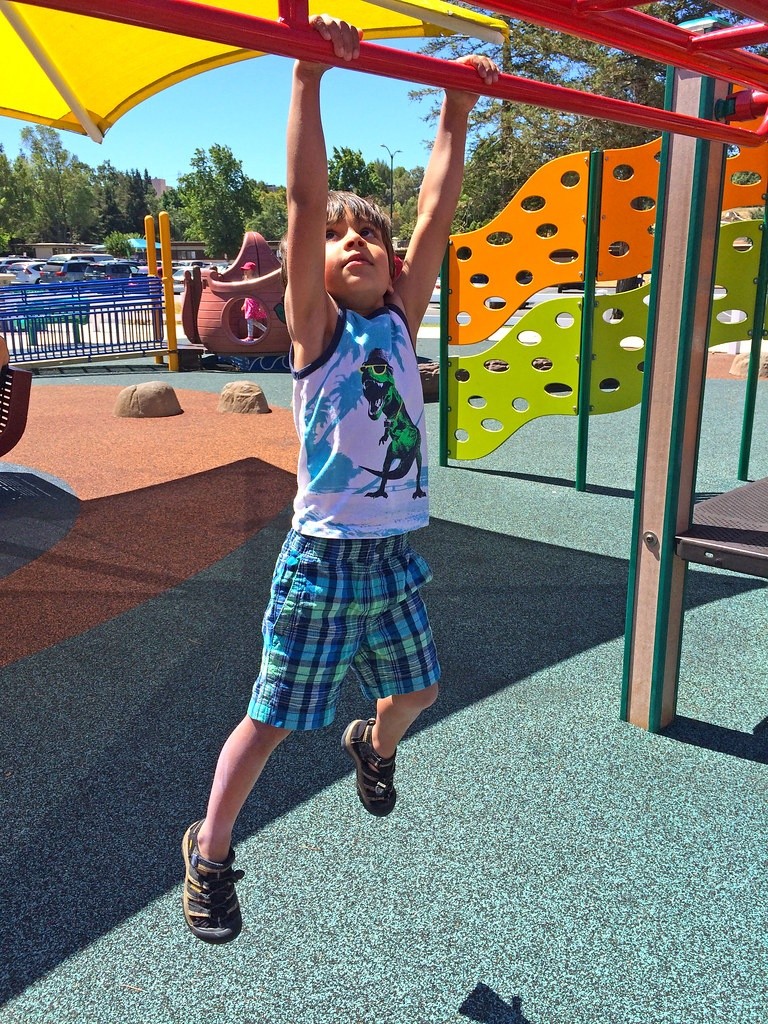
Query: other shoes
242,338,254,342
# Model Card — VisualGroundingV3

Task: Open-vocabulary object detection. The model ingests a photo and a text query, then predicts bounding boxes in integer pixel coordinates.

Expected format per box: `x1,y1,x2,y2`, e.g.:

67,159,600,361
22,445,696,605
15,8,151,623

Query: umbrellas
91,238,164,263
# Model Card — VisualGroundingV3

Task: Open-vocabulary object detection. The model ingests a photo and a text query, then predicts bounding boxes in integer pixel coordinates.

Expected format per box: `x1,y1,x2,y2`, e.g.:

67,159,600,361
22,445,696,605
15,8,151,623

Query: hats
240,262,256,271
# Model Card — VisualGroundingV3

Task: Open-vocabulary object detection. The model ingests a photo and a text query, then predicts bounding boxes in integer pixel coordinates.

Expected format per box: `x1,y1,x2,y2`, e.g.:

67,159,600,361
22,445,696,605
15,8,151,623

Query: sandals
179,818,245,943
341,717,397,817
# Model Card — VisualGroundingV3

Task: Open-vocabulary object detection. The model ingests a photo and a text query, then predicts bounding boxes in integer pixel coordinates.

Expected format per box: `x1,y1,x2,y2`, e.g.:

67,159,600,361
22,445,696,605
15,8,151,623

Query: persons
239,261,268,341
178,13,496,944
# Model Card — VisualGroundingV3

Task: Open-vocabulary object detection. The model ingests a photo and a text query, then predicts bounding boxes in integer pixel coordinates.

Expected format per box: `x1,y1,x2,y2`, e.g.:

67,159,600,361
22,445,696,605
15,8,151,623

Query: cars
0,253,231,294
